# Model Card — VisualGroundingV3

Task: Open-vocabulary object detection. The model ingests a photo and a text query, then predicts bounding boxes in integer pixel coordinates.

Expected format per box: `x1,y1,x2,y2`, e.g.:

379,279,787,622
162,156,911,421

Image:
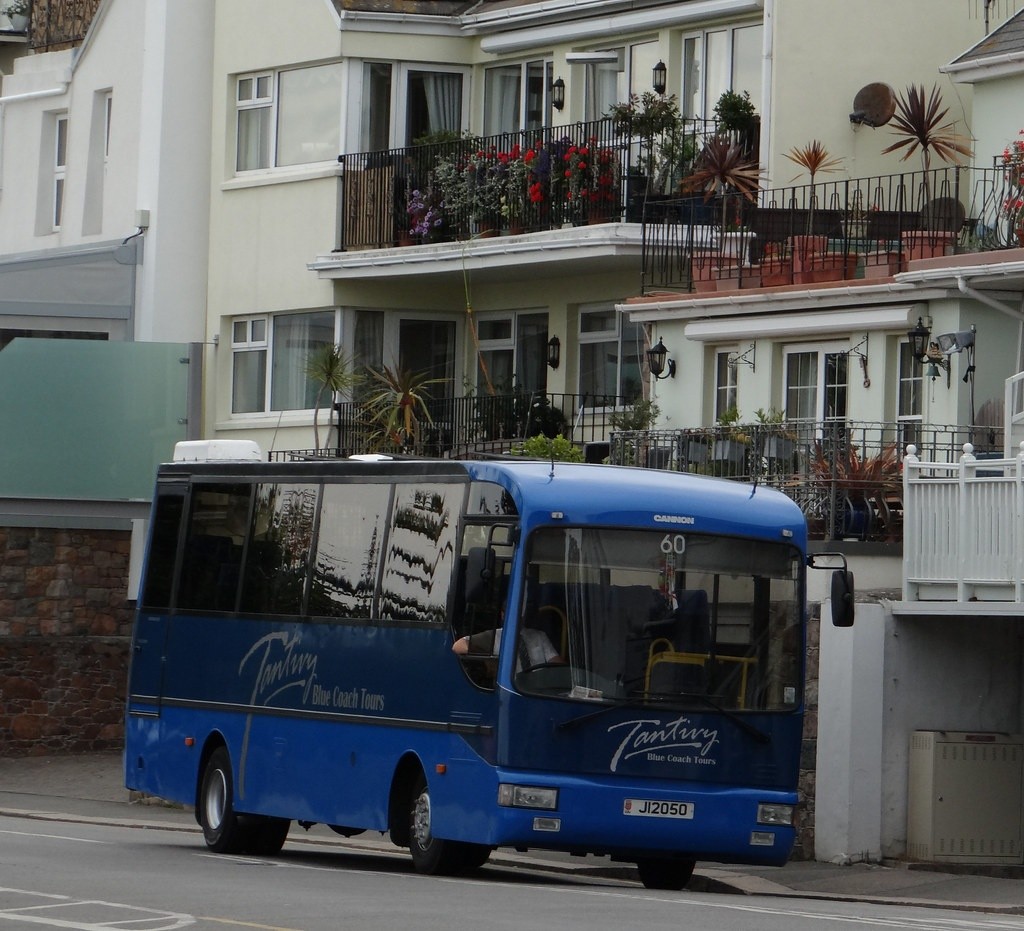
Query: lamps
553,76,565,110
646,336,675,382
546,334,560,371
907,316,950,402
653,59,666,94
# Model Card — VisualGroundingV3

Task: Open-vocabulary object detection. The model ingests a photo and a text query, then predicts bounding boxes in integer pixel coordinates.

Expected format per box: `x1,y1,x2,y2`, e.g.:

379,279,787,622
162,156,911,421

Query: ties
518,636,532,671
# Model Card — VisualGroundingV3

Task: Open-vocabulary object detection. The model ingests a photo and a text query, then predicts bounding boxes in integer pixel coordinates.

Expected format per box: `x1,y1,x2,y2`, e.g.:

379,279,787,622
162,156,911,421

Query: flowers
842,197,878,220
406,133,619,238
717,219,748,232
999,129,1024,248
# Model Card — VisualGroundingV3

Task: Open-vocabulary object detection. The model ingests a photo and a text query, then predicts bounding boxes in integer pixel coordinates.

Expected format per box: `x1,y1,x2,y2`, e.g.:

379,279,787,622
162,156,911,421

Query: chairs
175,534,291,614
624,617,677,698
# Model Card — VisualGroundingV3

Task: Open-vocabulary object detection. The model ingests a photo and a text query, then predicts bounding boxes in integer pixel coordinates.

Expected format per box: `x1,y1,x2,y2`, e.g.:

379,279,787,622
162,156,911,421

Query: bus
123,440,854,890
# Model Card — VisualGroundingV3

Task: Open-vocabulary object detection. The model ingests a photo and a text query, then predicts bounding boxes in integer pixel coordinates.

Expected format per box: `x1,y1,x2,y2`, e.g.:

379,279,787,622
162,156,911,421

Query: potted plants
601,88,760,223
393,175,416,247
808,438,902,534
881,84,974,272
782,140,845,284
674,408,796,463
683,132,768,293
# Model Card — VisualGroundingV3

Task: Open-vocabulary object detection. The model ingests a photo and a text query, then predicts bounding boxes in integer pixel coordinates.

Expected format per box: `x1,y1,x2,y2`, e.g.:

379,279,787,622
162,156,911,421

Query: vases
840,220,872,240
758,256,792,286
509,217,524,234
808,252,860,282
479,219,496,238
859,250,905,278
713,264,759,291
588,207,609,224
712,232,756,258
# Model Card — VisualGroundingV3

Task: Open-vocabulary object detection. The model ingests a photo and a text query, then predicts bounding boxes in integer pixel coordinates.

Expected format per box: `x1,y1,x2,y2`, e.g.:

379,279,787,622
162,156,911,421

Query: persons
452,598,568,673
